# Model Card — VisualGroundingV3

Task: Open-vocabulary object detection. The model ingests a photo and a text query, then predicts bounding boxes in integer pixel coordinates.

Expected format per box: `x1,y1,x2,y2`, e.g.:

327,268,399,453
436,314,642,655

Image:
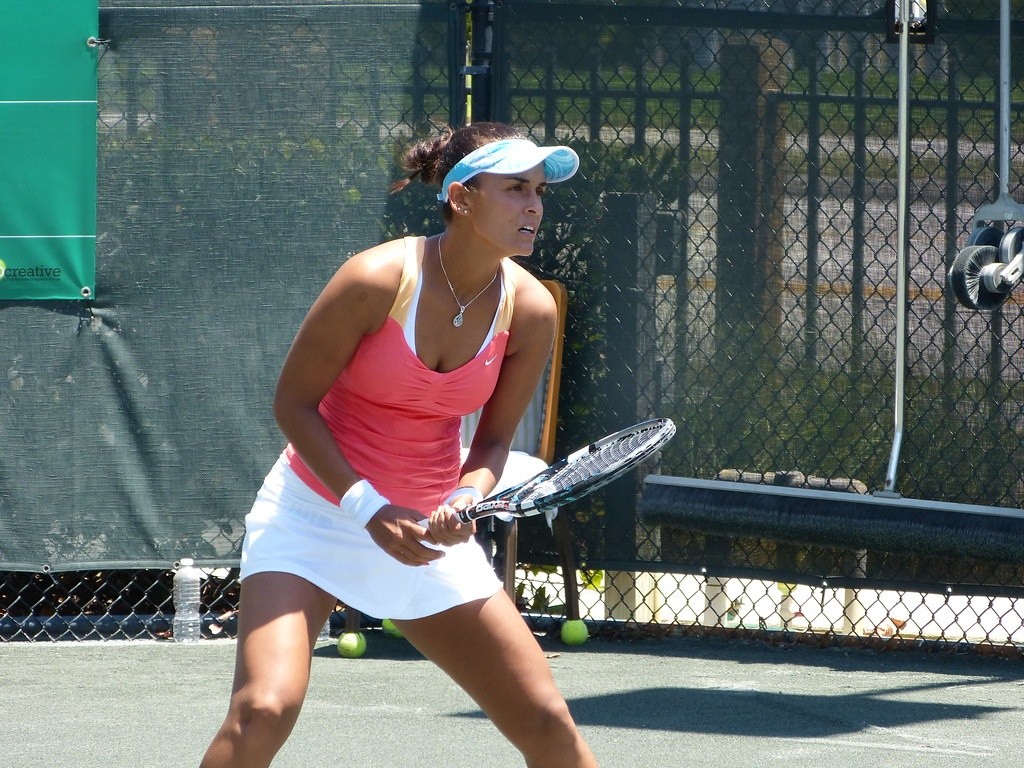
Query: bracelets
339,478,390,527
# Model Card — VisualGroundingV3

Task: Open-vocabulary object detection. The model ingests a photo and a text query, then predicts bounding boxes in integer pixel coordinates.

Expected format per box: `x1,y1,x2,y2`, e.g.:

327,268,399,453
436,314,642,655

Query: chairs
343,280,580,634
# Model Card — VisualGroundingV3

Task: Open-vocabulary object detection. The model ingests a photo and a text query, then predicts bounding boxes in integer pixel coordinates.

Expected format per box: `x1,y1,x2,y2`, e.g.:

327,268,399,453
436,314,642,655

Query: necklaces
439,233,498,327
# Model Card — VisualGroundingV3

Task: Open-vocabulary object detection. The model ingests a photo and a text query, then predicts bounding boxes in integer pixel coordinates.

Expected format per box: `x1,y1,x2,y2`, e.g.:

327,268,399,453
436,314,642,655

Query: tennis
561,620,588,644
337,631,366,658
381,617,404,639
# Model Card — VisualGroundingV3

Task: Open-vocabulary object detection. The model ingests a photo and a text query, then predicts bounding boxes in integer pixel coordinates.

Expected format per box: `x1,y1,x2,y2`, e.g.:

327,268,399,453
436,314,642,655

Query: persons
199,126,601,767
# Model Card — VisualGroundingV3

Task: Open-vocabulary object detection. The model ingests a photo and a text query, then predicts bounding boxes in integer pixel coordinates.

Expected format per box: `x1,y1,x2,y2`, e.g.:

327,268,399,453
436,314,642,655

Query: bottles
172,557,203,643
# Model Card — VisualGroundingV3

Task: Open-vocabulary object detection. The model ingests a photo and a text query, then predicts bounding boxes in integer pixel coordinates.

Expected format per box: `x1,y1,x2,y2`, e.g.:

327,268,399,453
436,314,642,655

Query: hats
436,138,580,204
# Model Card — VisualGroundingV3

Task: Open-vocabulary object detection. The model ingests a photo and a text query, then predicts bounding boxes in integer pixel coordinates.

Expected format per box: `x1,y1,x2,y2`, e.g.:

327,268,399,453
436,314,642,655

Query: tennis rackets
414,416,676,530
